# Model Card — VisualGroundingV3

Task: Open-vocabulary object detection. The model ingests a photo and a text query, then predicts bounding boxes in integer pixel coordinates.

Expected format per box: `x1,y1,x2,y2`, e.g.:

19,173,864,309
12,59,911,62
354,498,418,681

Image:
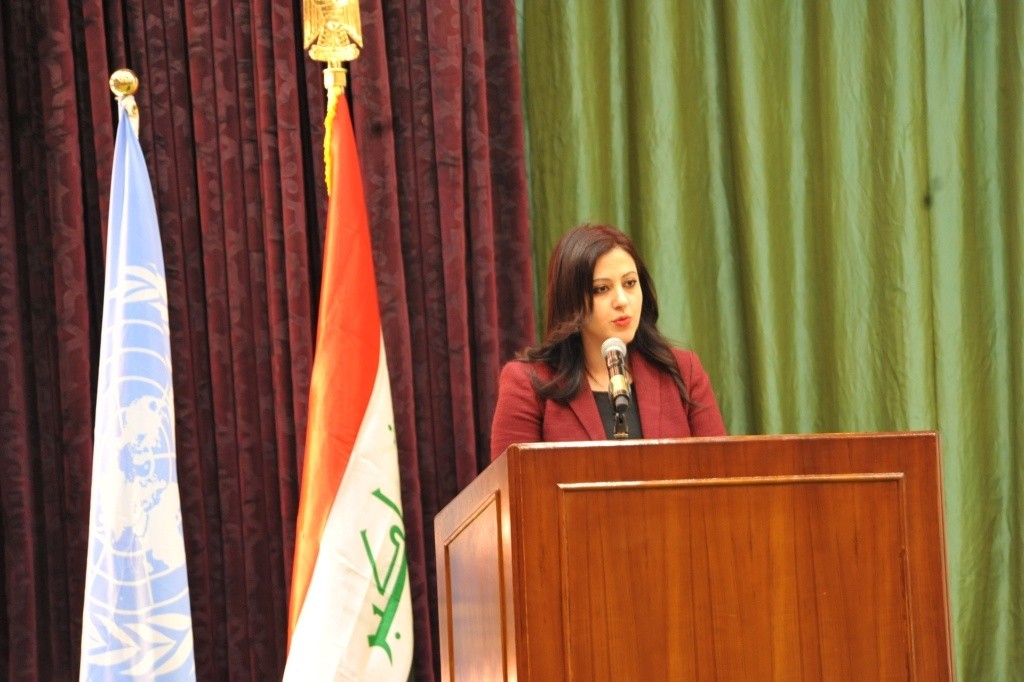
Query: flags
77,97,196,682
283,82,413,682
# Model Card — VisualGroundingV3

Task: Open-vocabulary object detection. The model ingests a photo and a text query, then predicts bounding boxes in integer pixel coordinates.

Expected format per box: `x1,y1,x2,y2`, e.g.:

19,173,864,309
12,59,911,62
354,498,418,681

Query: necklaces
586,359,629,391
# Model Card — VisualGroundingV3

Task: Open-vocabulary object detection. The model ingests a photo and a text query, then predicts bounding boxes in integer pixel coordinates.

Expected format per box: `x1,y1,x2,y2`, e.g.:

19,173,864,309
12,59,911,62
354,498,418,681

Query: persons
490,223,728,460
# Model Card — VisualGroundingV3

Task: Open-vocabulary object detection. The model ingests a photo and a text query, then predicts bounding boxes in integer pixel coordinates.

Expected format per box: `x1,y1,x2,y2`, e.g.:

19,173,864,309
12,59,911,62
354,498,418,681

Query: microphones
601,336,630,412
